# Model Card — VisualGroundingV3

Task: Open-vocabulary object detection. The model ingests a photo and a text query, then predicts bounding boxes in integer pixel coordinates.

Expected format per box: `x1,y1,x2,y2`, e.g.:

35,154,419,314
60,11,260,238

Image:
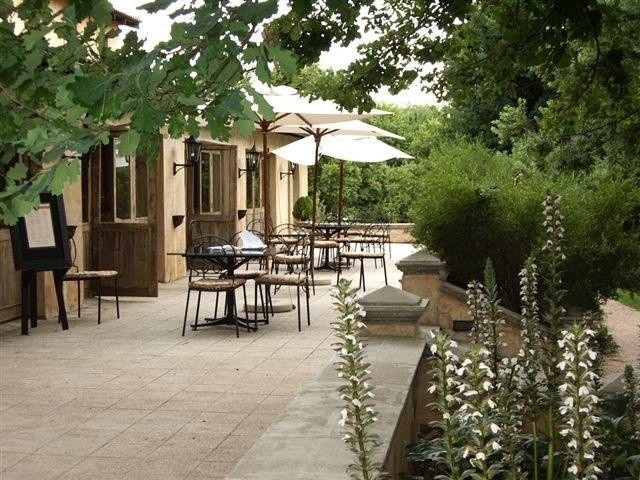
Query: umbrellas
271,135,415,251
182,80,395,239
271,119,407,273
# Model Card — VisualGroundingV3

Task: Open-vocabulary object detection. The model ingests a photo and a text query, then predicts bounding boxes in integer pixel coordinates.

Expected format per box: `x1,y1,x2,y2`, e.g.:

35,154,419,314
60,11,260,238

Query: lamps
172,134,202,175
280,160,297,181
239,139,262,177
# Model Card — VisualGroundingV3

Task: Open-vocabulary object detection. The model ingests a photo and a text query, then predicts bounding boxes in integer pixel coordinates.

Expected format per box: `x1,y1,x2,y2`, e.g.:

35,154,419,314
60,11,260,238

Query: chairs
57,236,120,324
181,209,391,339
